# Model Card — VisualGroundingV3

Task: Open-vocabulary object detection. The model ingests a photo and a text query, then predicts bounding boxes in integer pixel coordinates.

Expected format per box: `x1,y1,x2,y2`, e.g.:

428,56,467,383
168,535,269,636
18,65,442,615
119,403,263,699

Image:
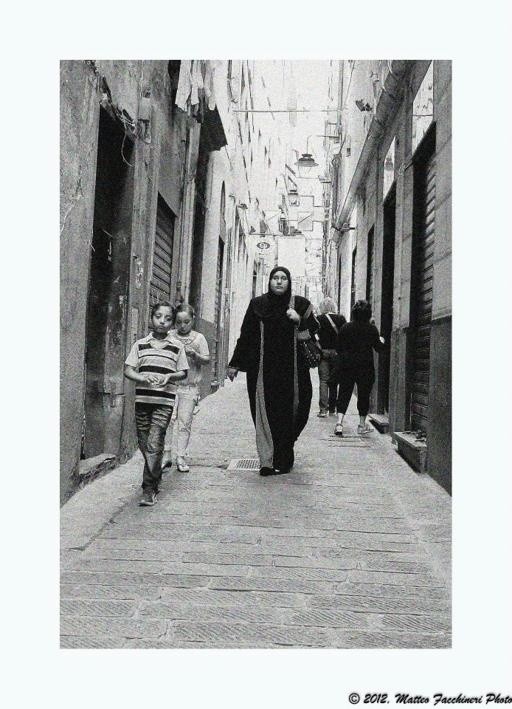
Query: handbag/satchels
297,328,323,369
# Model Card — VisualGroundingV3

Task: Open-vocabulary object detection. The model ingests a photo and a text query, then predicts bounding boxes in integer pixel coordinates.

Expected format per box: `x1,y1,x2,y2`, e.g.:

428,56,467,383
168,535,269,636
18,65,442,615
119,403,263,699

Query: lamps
295,133,341,169
286,175,335,206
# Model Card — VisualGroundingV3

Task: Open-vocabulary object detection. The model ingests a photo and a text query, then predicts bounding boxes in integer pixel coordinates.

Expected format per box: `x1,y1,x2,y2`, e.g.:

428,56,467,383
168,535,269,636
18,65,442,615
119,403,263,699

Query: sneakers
139,490,158,506
317,411,337,417
334,423,344,434
260,464,290,476
357,425,375,434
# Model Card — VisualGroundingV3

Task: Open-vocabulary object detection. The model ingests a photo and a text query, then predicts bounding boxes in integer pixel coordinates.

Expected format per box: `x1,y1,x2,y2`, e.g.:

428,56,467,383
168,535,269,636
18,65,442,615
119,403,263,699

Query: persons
316,297,347,417
124,301,189,506
161,304,210,471
227,267,321,476
332,300,385,436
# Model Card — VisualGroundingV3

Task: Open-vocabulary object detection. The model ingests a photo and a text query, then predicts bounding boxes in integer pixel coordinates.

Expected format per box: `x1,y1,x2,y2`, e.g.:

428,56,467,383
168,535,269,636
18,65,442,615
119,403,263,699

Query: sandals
161,461,172,473
176,458,190,472
